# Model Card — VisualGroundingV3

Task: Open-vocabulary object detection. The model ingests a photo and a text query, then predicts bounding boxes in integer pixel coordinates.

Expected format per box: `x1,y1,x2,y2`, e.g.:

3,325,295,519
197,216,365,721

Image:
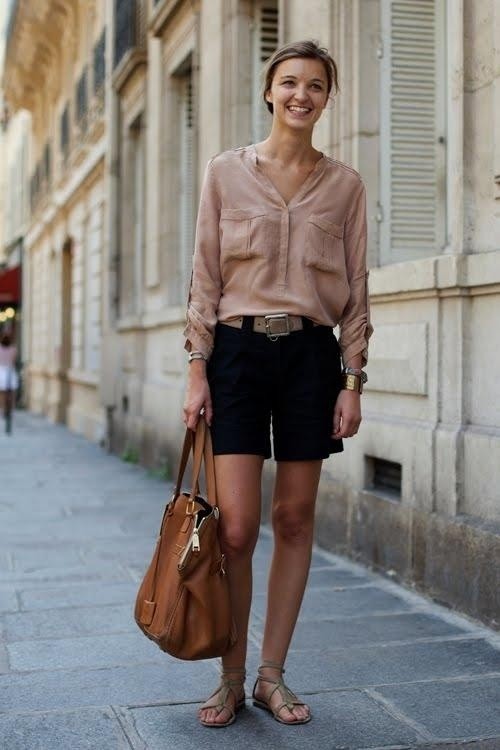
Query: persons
183,42,375,729
0,336,20,432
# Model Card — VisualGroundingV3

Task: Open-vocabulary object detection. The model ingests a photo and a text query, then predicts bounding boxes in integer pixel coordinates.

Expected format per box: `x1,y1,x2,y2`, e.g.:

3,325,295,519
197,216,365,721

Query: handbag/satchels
134,411,238,661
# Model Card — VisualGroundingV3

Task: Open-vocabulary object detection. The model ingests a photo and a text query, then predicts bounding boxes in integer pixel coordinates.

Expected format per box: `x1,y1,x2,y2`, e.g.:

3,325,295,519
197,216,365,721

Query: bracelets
342,374,364,395
188,351,203,361
343,368,368,384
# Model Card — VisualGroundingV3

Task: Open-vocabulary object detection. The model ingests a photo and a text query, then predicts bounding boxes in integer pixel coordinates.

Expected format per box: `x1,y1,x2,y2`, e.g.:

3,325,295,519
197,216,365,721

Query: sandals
252,662,311,725
199,666,247,728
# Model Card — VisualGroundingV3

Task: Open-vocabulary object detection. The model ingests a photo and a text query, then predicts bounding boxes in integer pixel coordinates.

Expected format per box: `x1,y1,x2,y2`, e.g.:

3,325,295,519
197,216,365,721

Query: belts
222,311,317,342
